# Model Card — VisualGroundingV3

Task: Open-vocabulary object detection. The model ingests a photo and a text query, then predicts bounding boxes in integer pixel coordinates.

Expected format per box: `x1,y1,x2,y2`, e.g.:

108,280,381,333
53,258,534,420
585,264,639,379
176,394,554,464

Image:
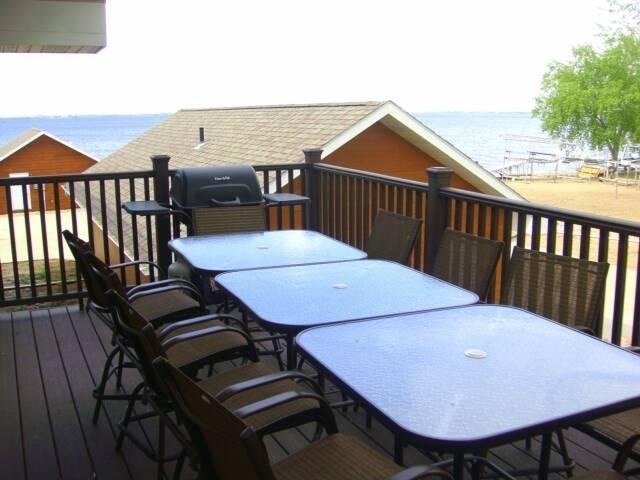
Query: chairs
62,203,640,479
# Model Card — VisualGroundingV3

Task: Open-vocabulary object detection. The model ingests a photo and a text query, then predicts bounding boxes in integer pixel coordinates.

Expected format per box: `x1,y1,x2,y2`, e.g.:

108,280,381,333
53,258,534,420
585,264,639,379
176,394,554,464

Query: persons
598,165,617,182
